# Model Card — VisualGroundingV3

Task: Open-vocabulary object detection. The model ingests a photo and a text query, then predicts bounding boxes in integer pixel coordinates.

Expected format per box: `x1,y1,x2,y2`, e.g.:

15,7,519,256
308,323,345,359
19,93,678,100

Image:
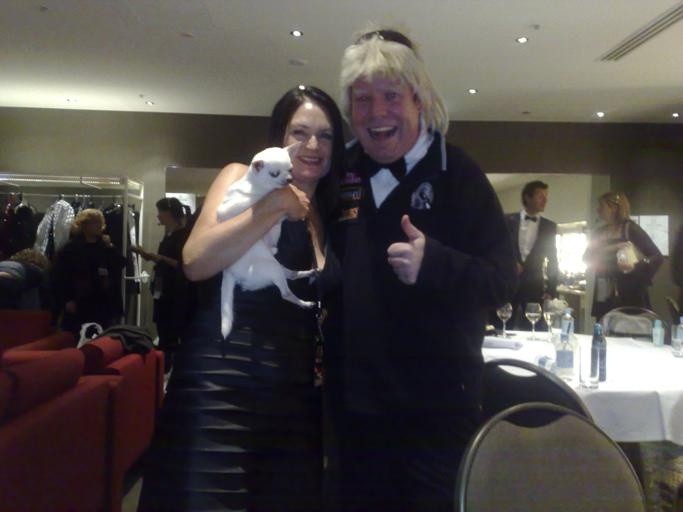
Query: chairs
0,309,165,510
599,305,665,340
458,359,646,511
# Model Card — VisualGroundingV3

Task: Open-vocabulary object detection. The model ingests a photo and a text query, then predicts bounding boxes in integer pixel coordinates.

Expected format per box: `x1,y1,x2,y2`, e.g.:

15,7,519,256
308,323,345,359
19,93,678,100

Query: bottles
561,308,575,335
592,324,606,382
536,354,553,370
677,317,683,337
652,320,665,346
556,335,573,377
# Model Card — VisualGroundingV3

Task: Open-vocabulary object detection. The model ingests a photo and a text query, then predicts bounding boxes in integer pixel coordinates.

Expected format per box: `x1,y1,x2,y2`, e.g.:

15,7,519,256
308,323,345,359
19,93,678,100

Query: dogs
215,140,317,340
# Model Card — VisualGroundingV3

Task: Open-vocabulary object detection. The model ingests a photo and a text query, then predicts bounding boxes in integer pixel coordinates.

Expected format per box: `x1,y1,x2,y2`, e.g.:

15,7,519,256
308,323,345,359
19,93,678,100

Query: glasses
356,30,412,49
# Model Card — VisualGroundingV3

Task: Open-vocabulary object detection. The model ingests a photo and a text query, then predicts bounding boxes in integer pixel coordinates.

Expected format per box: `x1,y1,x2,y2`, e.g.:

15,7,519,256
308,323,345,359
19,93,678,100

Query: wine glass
496,300,555,342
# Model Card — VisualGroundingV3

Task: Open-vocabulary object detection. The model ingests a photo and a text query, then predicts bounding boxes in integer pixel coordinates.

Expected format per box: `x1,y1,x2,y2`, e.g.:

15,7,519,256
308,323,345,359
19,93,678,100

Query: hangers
1,191,122,221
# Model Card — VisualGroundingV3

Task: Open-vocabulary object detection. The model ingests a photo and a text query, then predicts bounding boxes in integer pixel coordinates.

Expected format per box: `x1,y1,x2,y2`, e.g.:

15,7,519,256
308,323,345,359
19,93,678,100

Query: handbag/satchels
617,241,646,273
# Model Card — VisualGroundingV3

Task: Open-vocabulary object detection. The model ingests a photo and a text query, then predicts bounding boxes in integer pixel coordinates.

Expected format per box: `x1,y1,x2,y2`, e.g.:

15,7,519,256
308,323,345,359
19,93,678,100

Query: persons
11,248,59,323
131,197,193,374
582,190,664,323
136,85,346,512
490,180,558,332
311,30,510,512
57,205,125,331
670,226,683,323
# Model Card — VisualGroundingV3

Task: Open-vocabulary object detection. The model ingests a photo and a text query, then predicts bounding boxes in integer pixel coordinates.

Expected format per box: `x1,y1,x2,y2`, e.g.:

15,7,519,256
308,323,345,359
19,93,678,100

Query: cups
670,326,683,357
578,345,600,388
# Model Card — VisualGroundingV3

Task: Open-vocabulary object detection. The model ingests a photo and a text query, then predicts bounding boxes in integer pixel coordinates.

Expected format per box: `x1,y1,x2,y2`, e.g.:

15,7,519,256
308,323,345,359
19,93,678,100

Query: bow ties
362,153,406,180
525,215,536,222
